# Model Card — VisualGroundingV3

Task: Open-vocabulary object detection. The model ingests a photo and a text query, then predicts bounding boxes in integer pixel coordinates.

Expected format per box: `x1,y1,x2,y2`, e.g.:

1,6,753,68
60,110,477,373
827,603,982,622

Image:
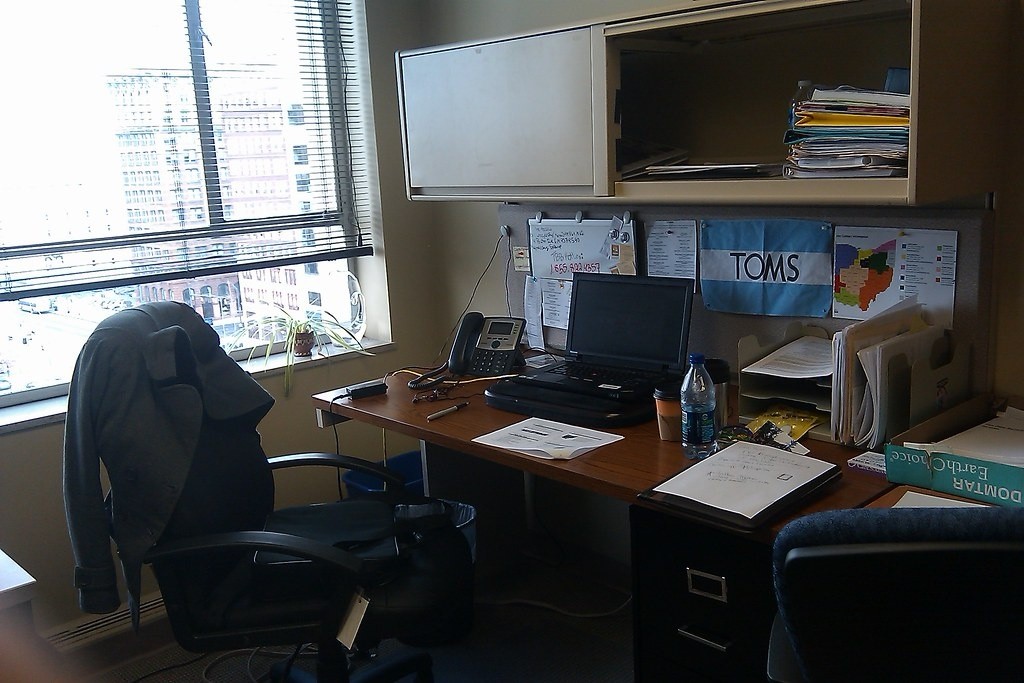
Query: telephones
447,311,528,376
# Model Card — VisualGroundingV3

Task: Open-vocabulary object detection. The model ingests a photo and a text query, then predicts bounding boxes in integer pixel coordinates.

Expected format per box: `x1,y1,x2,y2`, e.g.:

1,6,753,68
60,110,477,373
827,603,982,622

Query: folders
783,85,910,180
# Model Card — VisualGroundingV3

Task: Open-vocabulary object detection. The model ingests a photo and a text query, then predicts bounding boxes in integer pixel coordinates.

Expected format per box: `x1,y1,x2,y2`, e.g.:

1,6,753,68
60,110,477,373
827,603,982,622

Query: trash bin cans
390,497,479,648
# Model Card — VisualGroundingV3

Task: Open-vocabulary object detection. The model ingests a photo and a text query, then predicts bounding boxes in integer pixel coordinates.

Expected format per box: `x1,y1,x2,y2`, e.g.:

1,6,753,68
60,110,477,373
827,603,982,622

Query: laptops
510,272,695,399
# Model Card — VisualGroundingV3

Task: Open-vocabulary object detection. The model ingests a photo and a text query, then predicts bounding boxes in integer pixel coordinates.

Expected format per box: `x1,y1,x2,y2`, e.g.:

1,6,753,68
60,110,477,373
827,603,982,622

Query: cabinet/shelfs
391,0,1003,206
737,322,837,445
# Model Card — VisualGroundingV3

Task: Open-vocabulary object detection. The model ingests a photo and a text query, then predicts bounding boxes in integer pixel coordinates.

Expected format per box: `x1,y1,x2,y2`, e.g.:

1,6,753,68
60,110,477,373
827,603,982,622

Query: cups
654,384,682,441
702,358,730,429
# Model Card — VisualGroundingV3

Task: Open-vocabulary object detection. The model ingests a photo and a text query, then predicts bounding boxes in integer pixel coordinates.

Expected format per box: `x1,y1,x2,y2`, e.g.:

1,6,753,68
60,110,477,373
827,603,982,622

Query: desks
311,341,895,540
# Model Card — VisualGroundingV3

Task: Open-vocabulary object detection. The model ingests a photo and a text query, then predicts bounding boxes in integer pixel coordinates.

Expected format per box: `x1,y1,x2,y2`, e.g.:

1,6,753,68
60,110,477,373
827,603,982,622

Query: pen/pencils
427,402,471,423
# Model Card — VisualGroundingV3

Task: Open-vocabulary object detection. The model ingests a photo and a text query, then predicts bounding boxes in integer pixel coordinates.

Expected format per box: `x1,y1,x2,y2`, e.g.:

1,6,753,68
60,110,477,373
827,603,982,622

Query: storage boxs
884,396,1024,505
341,450,422,502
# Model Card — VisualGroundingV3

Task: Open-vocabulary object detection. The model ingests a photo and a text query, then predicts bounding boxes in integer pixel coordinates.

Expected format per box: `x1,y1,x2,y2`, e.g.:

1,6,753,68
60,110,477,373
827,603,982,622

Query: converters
346,381,387,400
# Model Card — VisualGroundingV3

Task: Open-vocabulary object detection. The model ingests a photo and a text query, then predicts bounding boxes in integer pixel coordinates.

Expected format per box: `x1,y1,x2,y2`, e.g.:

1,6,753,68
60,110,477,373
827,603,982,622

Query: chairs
62,301,478,683
765,506,1023,682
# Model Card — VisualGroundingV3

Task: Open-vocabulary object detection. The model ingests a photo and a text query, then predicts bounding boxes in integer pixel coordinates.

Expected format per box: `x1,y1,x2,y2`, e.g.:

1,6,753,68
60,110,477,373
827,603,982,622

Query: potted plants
219,296,374,403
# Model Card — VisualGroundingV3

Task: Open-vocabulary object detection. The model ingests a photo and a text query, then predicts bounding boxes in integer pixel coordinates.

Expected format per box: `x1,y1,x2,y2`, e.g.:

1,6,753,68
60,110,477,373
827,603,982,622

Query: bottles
788,80,814,129
679,352,717,461
